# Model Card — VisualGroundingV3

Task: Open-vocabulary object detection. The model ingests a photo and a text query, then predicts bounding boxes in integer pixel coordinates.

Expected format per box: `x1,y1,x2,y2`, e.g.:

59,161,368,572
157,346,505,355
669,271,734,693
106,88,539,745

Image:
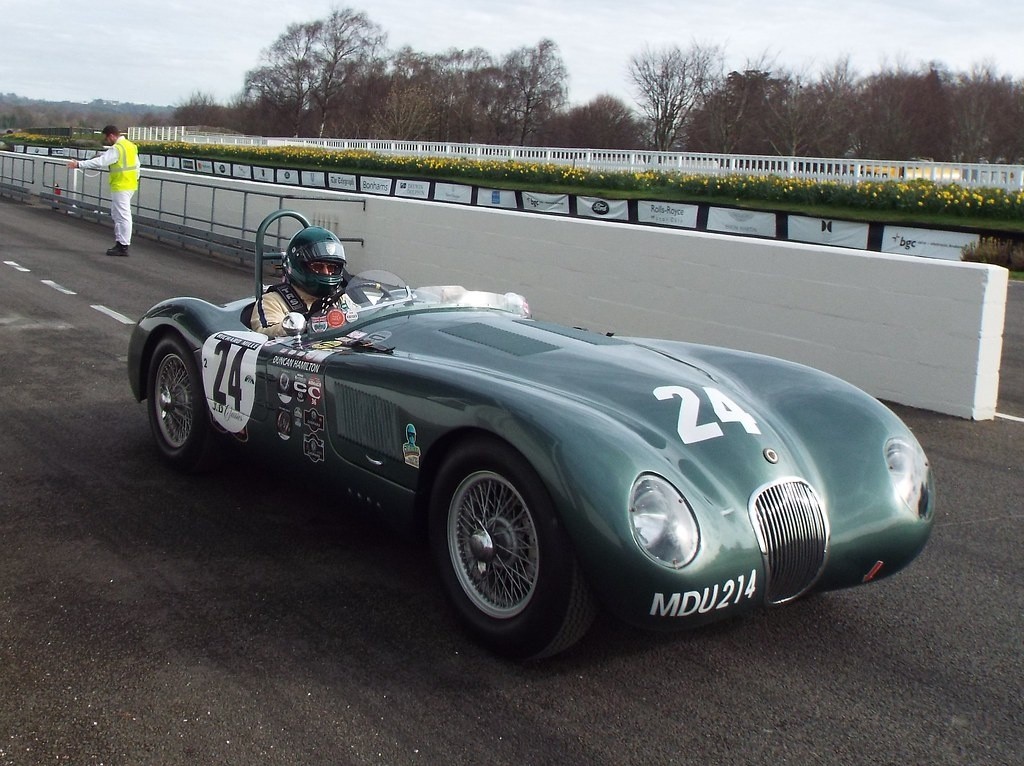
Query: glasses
105,136,108,142
309,261,339,273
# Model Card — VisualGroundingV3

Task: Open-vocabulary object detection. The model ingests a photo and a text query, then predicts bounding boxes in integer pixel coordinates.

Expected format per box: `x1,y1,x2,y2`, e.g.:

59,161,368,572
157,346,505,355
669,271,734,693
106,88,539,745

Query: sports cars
125,208,940,664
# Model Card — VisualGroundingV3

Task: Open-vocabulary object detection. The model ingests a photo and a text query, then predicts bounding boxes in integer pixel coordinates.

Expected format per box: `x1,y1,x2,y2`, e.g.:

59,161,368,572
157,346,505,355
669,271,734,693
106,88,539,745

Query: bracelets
76,160,80,169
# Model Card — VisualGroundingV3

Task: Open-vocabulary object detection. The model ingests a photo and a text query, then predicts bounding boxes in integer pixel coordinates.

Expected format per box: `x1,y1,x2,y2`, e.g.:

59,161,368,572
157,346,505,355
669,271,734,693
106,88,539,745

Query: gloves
302,296,333,322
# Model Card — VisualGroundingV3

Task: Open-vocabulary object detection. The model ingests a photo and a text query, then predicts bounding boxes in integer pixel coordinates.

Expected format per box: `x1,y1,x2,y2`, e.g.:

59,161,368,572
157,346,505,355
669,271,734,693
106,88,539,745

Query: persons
250,226,363,340
67,125,140,256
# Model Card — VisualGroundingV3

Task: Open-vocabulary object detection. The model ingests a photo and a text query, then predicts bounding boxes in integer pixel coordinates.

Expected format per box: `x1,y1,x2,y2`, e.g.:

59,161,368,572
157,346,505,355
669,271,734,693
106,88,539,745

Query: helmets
285,226,347,296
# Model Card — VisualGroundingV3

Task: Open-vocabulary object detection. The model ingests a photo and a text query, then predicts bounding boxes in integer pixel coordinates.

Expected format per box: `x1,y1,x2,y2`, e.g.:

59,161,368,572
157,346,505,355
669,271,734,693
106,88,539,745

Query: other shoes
106,241,128,257
